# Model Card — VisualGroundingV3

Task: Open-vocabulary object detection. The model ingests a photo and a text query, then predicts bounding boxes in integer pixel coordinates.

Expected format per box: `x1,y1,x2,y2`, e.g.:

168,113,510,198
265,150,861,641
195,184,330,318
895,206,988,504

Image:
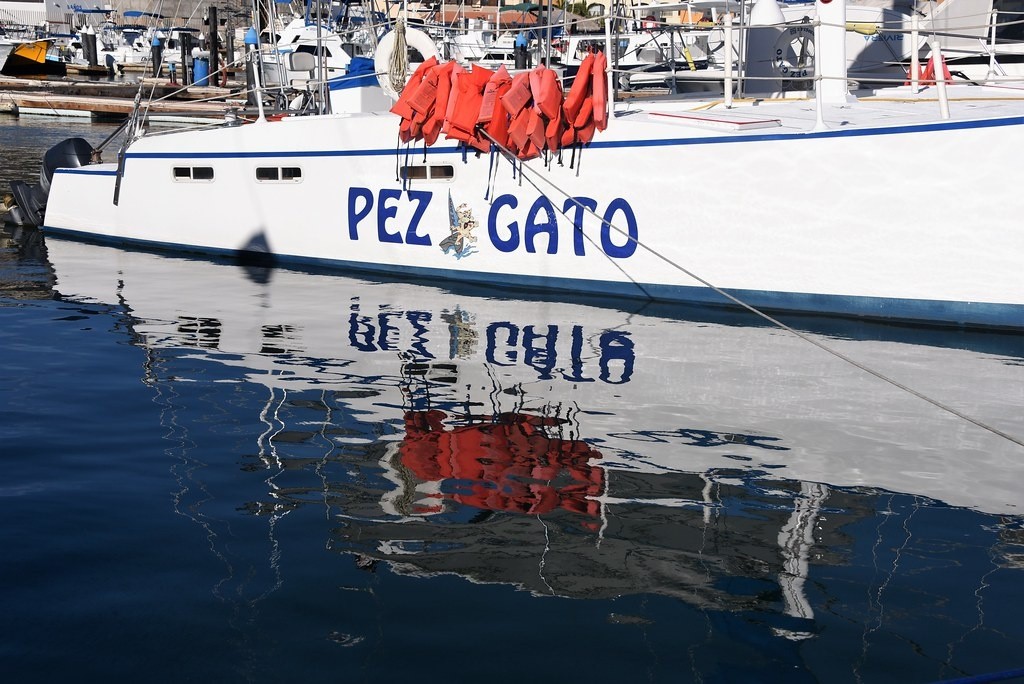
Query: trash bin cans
192,58,209,86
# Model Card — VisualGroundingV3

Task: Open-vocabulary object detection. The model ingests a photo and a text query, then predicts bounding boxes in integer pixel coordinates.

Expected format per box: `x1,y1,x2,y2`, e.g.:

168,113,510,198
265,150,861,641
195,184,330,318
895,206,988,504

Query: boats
0,0,1024,329
8,228,1024,641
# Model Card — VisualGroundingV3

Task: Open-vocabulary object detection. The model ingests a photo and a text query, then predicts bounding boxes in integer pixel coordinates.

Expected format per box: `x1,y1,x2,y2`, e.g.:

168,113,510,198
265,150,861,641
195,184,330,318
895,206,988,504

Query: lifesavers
773,25,816,77
697,18,710,30
374,27,440,102
642,16,657,33
